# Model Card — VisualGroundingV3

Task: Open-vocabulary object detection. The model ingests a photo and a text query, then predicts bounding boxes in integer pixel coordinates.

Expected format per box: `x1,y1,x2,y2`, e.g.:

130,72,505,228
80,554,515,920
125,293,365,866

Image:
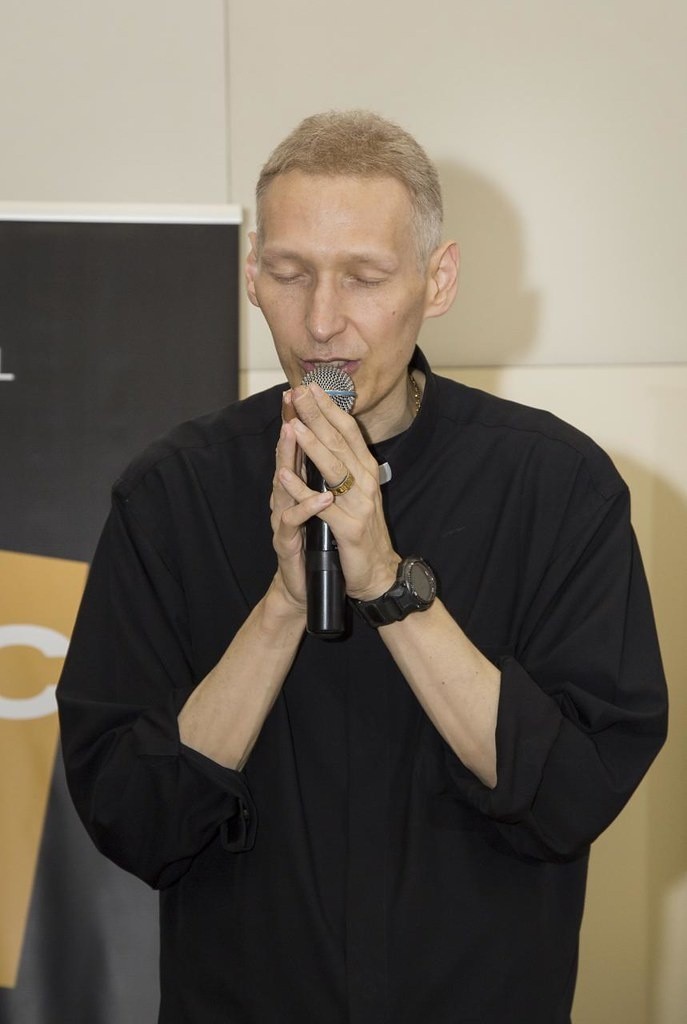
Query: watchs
347,546,438,630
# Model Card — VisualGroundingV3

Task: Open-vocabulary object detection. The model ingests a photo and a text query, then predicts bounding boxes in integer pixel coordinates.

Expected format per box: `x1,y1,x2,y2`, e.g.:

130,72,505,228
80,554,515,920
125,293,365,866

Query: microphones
302,366,359,640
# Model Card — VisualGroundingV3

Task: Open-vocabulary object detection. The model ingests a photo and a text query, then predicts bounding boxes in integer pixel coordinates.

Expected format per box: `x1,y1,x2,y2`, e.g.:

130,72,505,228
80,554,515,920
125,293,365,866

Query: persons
54,109,667,1023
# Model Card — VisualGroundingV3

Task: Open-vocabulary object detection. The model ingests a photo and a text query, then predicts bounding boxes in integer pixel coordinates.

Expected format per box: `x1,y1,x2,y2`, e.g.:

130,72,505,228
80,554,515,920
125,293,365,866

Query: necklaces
405,363,423,419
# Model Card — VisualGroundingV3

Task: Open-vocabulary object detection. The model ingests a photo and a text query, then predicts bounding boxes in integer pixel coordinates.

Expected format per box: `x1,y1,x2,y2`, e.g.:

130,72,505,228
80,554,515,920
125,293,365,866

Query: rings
324,470,357,497
